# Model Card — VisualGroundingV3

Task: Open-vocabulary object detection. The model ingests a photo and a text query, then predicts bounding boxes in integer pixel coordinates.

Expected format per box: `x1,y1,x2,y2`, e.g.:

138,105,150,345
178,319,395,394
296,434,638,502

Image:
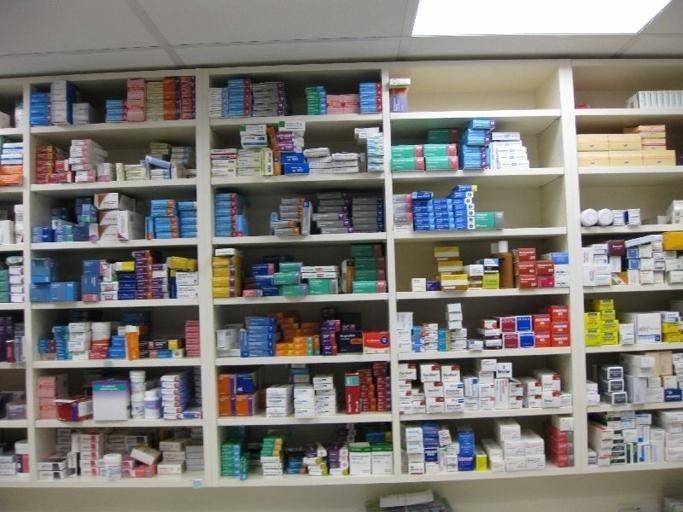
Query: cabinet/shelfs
1,58,683,488
